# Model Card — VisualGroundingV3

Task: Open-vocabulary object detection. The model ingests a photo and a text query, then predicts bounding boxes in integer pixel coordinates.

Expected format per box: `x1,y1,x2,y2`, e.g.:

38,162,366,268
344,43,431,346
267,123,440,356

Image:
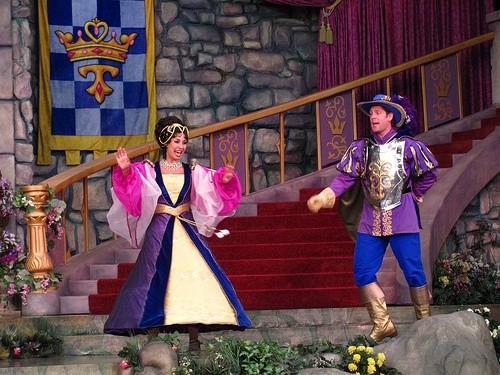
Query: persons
307,94,439,345
104,115,254,360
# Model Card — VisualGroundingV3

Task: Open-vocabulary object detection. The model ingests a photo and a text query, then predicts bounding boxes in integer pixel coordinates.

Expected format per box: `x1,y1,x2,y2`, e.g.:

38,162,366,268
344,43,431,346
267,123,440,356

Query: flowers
0,169,68,308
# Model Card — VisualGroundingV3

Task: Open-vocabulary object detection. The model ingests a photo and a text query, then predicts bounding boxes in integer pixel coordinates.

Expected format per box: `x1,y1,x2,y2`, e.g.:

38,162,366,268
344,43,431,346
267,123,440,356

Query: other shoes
188,339,203,354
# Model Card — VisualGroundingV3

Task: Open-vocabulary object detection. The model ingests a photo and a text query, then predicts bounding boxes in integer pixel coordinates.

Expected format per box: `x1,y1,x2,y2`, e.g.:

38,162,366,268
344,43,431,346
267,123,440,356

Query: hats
356,93,407,128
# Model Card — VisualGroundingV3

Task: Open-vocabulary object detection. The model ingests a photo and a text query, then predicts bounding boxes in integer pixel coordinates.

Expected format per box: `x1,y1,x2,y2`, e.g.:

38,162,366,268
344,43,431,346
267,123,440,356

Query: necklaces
160,158,183,171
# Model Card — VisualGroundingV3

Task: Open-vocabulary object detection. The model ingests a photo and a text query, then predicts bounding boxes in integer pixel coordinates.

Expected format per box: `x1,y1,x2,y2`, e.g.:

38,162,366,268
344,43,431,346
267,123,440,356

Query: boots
360,281,398,344
409,284,431,320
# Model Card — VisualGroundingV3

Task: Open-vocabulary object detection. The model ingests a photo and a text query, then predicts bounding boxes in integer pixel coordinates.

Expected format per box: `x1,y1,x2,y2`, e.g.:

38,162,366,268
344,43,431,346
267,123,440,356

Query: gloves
307,187,335,213
